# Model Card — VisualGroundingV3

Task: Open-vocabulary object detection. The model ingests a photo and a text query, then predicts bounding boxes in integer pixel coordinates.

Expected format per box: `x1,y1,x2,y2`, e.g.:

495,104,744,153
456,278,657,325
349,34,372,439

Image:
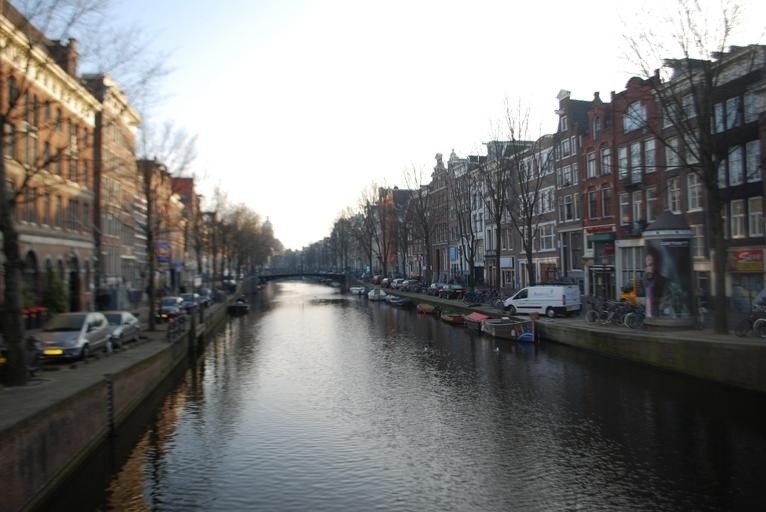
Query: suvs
30,310,116,364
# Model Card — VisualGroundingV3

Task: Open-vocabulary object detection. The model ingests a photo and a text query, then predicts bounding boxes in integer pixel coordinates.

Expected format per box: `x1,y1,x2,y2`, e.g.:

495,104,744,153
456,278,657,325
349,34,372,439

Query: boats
480,318,537,343
227,294,251,315
464,311,491,332
440,309,464,323
348,284,368,295
416,303,437,314
367,288,387,302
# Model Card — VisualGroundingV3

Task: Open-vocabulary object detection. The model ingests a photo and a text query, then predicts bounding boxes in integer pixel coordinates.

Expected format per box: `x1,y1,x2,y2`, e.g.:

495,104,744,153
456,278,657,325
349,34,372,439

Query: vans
502,284,582,320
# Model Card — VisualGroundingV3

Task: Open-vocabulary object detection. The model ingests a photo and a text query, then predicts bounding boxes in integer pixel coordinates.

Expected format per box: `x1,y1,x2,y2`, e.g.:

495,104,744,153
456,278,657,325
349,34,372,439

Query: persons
646,253,670,318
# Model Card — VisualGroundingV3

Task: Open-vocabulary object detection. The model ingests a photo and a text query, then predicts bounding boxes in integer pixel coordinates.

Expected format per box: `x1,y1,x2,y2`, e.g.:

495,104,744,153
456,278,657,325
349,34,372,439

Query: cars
370,274,520,306
147,275,238,322
99,309,144,350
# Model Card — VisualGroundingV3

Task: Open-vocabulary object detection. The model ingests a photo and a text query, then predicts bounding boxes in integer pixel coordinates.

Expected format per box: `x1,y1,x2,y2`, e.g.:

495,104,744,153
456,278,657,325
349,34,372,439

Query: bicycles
586,295,765,340
